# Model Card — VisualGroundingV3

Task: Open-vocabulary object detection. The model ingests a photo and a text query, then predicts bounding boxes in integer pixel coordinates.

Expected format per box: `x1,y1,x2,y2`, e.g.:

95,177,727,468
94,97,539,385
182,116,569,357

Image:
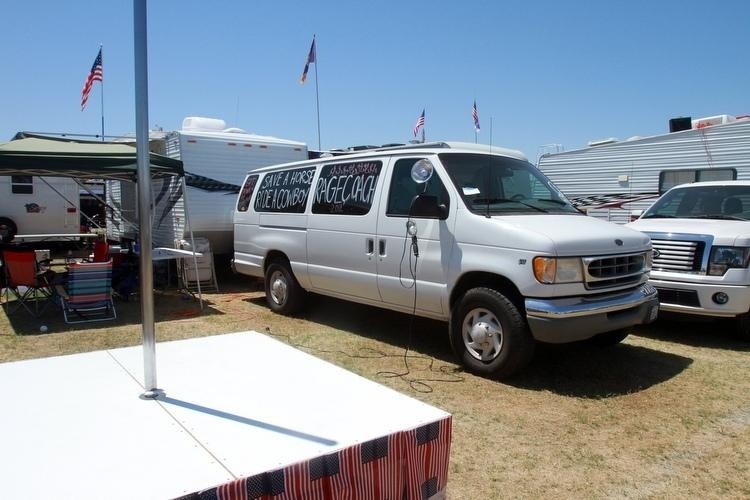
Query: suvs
621,178,750,331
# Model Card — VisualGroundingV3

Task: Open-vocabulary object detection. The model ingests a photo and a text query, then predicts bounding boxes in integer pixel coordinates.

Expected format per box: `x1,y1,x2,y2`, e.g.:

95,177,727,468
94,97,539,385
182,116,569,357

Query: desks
134,247,204,303
13,233,98,259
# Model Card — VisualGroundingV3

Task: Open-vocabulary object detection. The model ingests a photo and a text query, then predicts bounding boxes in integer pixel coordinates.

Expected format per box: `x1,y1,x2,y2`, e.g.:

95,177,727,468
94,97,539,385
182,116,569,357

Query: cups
133,244,140,252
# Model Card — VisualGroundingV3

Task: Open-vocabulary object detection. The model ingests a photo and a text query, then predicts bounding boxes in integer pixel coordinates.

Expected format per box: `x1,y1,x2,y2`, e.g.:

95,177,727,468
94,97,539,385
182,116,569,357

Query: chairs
2,250,58,319
60,260,118,324
699,194,742,218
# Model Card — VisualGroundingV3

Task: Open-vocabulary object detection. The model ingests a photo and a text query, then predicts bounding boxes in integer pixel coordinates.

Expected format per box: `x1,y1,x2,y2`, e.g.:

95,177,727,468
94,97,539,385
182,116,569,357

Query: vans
230,139,660,378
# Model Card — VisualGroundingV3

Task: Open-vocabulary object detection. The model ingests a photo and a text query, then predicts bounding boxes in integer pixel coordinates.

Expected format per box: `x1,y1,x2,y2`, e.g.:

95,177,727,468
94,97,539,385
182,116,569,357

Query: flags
79,47,103,113
470,97,480,133
412,108,425,138
299,37,317,87
